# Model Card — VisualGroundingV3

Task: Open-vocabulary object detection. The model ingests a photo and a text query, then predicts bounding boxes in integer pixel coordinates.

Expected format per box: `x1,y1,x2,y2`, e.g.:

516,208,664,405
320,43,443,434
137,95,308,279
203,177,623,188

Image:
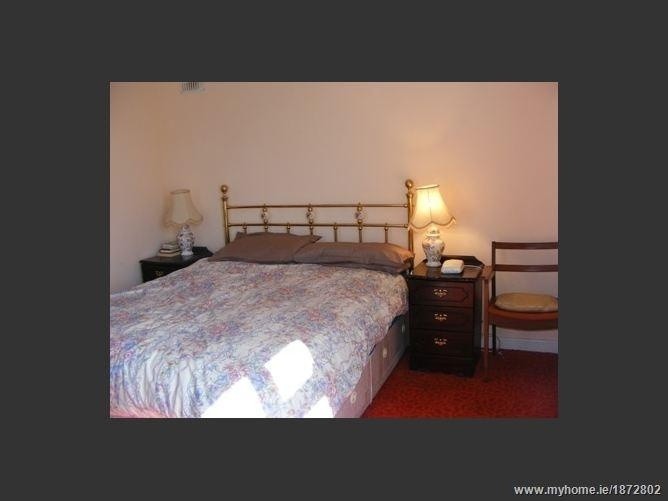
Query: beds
110,180,415,419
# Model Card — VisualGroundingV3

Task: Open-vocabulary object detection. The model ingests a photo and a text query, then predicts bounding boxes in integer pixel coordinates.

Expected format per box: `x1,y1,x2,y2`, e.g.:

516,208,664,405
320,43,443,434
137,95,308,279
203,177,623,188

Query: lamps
407,184,457,266
164,188,204,256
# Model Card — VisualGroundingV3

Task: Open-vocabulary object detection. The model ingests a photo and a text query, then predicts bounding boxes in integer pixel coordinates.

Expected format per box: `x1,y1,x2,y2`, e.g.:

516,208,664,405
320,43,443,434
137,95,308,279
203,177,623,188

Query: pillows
207,232,415,274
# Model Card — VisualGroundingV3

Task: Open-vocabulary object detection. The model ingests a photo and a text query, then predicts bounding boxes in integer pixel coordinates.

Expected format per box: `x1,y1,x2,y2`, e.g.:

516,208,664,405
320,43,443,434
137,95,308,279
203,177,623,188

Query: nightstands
139,246,214,282
405,255,485,377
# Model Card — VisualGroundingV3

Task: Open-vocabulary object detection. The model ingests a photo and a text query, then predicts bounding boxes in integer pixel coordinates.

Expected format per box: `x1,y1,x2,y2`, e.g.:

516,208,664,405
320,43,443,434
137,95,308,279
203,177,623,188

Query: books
161,241,181,250
160,249,181,254
157,251,181,258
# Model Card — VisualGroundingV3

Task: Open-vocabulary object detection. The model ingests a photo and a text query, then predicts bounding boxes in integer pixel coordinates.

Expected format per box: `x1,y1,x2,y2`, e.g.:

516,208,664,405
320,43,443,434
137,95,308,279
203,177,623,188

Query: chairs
485,241,558,382
